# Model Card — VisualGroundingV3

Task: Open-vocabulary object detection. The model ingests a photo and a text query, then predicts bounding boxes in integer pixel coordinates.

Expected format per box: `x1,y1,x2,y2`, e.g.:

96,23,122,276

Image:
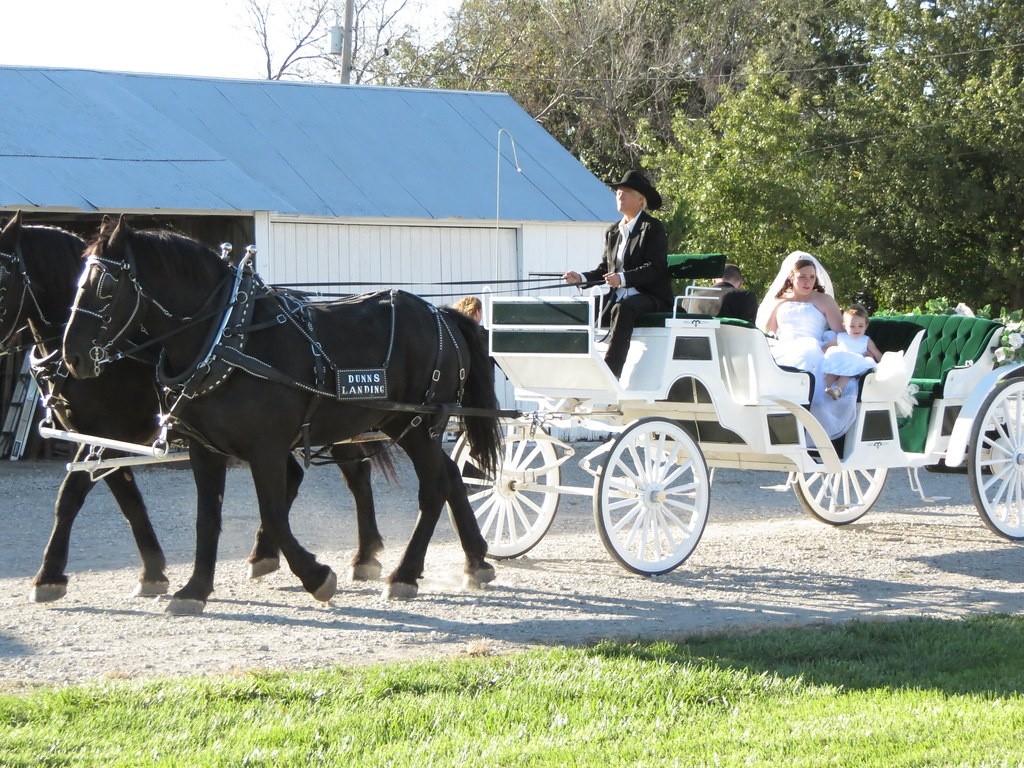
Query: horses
59,214,506,618
0,209,406,603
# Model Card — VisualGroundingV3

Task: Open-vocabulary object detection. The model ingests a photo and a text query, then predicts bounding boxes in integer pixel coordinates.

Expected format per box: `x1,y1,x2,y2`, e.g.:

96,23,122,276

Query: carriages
0,211,1024,624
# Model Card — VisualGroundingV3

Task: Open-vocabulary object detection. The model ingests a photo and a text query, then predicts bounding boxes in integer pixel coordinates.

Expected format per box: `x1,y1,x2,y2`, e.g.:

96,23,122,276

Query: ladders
0,343,59,462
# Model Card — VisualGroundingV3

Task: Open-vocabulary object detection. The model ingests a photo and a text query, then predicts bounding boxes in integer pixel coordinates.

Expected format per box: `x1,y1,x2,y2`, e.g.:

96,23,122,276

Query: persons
681,263,759,328
561,169,677,388
753,251,870,448
820,303,882,401
456,294,494,490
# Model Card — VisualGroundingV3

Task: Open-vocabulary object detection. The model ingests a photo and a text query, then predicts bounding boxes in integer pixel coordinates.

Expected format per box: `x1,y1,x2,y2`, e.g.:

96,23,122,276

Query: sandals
825,386,842,401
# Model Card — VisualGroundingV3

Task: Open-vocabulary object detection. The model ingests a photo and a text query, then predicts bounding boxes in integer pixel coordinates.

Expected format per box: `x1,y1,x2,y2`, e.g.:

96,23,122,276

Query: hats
603,170,662,210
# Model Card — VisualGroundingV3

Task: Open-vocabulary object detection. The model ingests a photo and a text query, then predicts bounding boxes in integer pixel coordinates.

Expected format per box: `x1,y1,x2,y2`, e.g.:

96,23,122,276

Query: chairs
848,315,926,402
711,313,812,407
866,315,1003,400
593,254,728,333
